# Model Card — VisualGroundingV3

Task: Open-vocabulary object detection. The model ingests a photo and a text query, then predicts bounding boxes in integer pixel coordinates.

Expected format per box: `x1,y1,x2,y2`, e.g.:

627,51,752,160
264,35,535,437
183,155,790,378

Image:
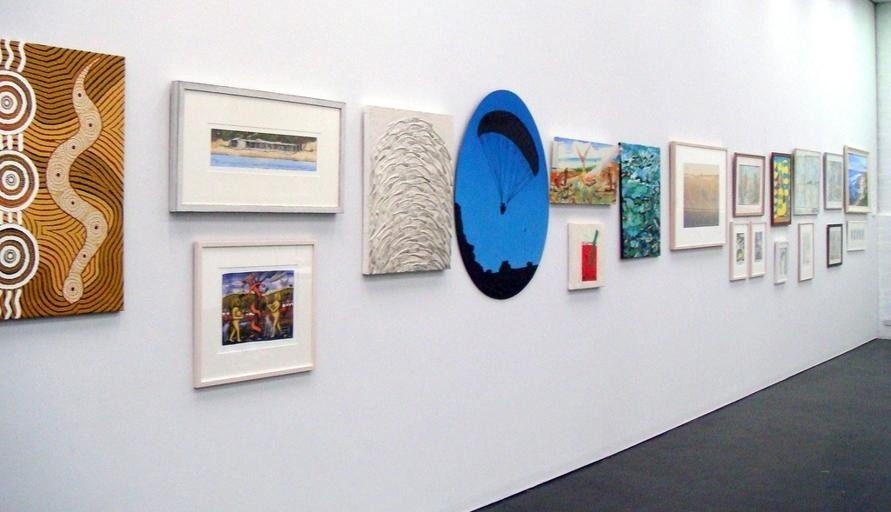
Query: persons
228,298,244,343
608,166,613,191
266,293,283,338
563,168,569,186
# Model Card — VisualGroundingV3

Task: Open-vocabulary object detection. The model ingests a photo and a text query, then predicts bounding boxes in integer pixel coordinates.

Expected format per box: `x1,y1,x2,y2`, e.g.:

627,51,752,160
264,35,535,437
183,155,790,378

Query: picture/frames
168,80,347,214
669,139,871,285
192,239,318,389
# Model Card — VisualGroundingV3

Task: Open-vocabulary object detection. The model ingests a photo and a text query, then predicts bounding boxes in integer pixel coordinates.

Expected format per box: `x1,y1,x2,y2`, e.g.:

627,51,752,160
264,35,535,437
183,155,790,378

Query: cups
580,240,598,282
